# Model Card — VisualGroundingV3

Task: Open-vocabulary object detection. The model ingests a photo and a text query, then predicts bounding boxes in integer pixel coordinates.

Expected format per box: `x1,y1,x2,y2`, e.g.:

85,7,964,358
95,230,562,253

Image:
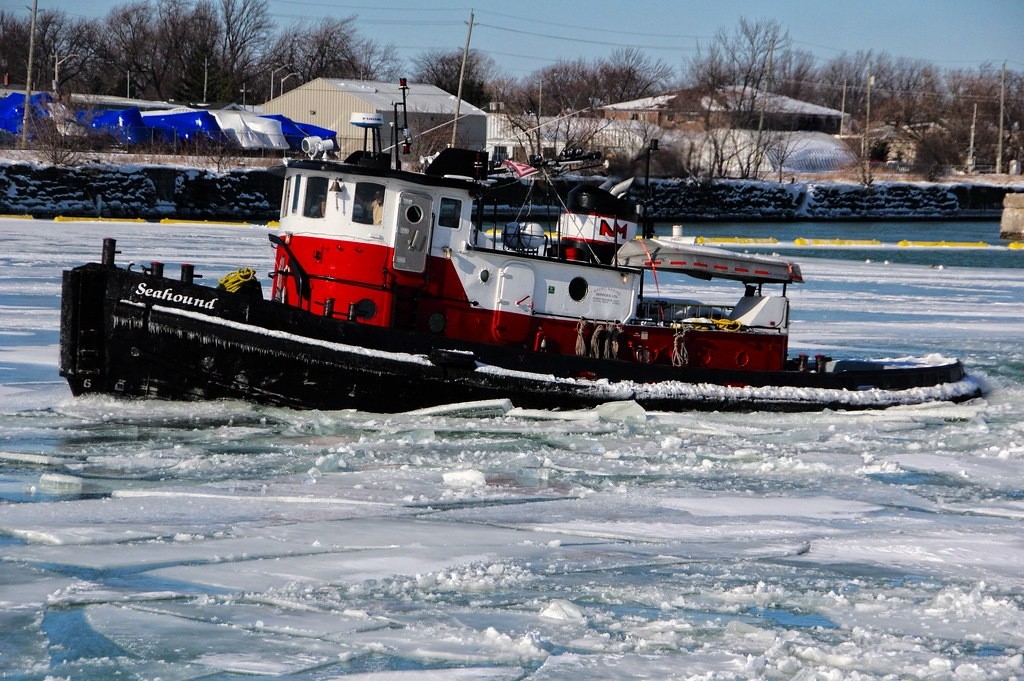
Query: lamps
620,272,630,285
442,245,452,260
330,177,344,194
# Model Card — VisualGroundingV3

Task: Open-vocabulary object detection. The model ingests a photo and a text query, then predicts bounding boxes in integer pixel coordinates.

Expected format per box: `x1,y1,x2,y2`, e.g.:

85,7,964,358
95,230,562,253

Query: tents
0,92,340,157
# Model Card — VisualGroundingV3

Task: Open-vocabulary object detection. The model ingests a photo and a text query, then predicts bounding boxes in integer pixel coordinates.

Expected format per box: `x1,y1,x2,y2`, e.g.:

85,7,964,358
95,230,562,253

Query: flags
502,158,538,177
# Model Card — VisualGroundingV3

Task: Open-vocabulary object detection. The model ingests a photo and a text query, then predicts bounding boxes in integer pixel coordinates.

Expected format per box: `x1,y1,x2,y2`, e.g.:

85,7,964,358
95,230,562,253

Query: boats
58,77,983,418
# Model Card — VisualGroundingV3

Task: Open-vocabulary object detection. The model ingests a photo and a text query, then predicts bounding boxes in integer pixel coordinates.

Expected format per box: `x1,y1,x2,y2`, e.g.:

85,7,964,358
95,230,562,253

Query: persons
355,188,383,226
46,90,128,153
4,72,8,88
